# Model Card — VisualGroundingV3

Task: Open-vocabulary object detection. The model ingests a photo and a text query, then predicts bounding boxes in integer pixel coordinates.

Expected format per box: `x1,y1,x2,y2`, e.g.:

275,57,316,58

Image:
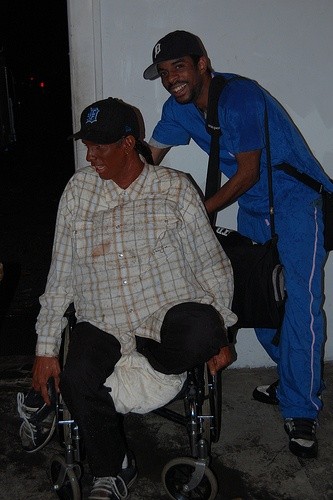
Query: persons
145,29,333,459
31,95,238,500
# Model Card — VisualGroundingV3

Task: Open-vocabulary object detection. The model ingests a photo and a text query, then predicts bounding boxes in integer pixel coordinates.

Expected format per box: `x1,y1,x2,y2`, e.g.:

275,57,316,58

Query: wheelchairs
48,303,223,499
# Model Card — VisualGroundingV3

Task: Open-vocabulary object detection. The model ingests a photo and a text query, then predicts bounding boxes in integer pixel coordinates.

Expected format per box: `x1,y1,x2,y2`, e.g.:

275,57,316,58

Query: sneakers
88,458,138,500
17,384,59,454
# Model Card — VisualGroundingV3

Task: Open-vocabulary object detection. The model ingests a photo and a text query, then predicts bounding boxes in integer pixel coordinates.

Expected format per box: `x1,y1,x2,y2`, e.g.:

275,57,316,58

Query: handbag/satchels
211,225,288,347
321,179,333,254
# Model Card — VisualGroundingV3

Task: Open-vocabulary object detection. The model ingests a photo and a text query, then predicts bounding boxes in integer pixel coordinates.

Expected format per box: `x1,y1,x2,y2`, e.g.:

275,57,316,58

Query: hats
143,30,206,80
67,97,140,144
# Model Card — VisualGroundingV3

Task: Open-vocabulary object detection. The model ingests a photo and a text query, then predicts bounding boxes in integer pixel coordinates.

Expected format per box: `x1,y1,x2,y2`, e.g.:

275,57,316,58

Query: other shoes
284,417,318,458
254,378,322,406
